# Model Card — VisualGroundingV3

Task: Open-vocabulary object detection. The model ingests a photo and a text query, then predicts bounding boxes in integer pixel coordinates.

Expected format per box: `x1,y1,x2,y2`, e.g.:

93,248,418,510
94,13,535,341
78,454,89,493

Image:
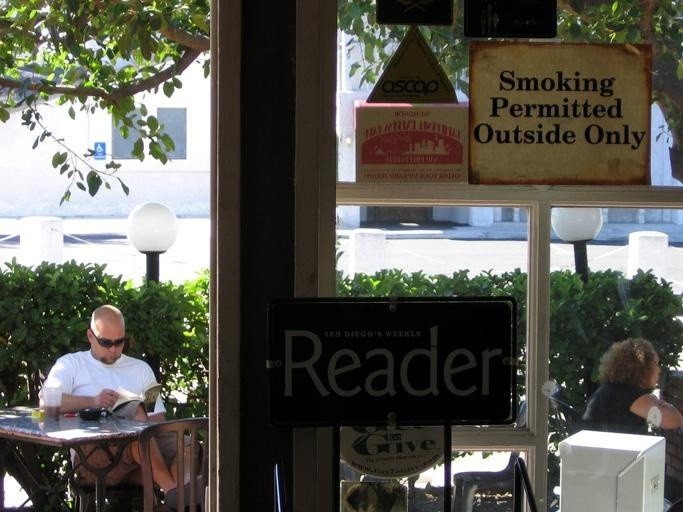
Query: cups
43,388,62,419
43,417,60,436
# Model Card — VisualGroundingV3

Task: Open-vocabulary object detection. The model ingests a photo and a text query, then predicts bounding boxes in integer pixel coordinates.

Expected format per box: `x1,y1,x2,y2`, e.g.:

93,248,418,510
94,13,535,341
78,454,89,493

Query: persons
36,304,201,510
581,337,683,438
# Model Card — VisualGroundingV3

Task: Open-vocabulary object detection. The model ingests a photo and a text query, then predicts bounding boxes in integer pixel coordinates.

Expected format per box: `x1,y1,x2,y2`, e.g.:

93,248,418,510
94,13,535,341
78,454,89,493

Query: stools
68,482,144,510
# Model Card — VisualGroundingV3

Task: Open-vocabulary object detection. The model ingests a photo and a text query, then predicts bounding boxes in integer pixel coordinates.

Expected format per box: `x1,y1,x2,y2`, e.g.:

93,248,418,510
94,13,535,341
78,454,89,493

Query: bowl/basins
78,408,103,420
80,421,101,429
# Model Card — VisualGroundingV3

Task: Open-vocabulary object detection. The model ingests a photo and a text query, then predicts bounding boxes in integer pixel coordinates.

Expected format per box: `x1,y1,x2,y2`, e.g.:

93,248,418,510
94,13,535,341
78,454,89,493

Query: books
108,383,161,413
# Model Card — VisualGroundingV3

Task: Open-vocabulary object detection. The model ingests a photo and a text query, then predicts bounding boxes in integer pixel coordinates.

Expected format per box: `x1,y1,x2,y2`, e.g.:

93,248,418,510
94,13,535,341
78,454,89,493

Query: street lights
550,205,605,285
124,201,180,284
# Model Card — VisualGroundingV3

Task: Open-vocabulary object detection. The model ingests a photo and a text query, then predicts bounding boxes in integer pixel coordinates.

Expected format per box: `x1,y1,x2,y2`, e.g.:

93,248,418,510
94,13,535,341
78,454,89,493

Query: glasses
90,328,126,348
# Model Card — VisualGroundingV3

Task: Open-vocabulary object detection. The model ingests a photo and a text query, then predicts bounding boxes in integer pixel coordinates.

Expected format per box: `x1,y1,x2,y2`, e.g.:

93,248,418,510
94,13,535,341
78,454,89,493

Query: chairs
139,417,208,510
546,394,681,511
451,393,527,511
